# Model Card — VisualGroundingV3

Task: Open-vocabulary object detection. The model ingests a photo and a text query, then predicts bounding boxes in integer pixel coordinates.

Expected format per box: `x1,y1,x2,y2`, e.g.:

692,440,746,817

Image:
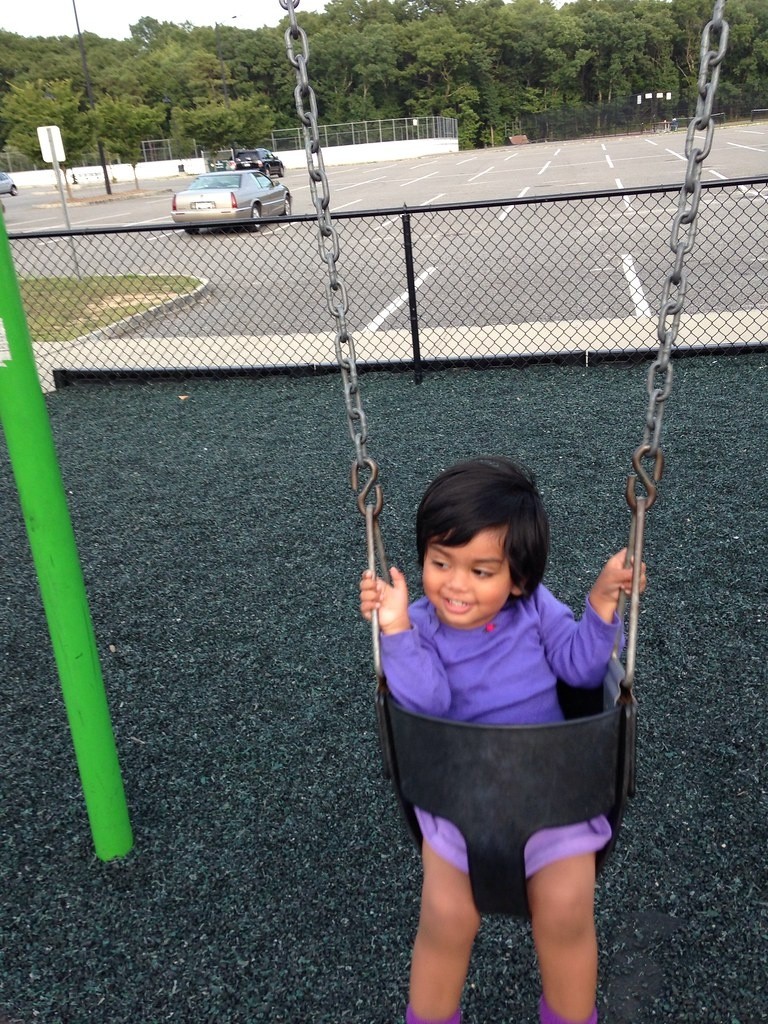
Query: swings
279,0,729,917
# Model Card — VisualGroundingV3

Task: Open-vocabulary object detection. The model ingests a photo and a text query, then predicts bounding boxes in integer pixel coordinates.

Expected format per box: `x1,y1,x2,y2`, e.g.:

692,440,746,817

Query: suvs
228,147,285,178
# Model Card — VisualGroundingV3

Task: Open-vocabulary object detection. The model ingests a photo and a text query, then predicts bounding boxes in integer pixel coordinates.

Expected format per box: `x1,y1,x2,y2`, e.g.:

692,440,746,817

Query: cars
0,171,20,197
171,171,293,233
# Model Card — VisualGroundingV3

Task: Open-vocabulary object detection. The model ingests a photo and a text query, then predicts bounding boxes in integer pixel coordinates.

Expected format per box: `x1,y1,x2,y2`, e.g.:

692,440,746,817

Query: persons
360,457,646,1023
